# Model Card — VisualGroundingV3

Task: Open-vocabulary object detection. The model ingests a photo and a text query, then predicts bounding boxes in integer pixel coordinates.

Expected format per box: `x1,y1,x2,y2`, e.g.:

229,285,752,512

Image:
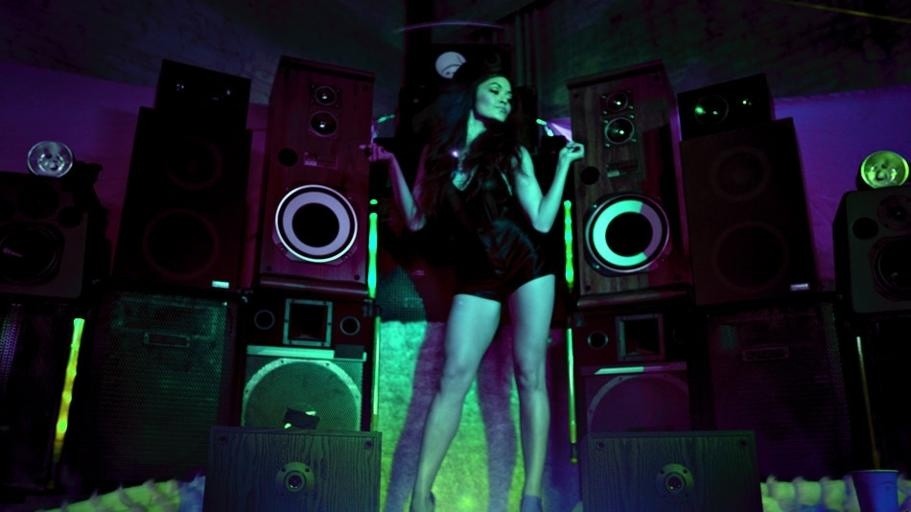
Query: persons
360,63,586,512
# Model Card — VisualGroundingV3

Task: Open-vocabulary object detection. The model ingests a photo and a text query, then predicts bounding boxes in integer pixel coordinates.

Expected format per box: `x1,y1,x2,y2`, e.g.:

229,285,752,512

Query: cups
851,470,901,512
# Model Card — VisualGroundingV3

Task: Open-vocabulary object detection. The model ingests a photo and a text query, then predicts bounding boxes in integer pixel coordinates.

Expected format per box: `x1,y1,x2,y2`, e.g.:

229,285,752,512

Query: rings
365,147,373,158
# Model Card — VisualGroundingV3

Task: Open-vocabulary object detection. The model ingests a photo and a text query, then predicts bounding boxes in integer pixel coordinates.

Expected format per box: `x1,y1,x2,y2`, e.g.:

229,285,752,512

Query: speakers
680,115,820,302
209,426,380,512
844,321,910,479
110,57,251,292
90,296,225,486
576,430,759,511
253,56,375,299
707,304,837,478
1,306,86,511
238,298,374,431
569,315,694,433
675,73,781,137
1,173,92,302
564,59,695,296
829,188,911,314
